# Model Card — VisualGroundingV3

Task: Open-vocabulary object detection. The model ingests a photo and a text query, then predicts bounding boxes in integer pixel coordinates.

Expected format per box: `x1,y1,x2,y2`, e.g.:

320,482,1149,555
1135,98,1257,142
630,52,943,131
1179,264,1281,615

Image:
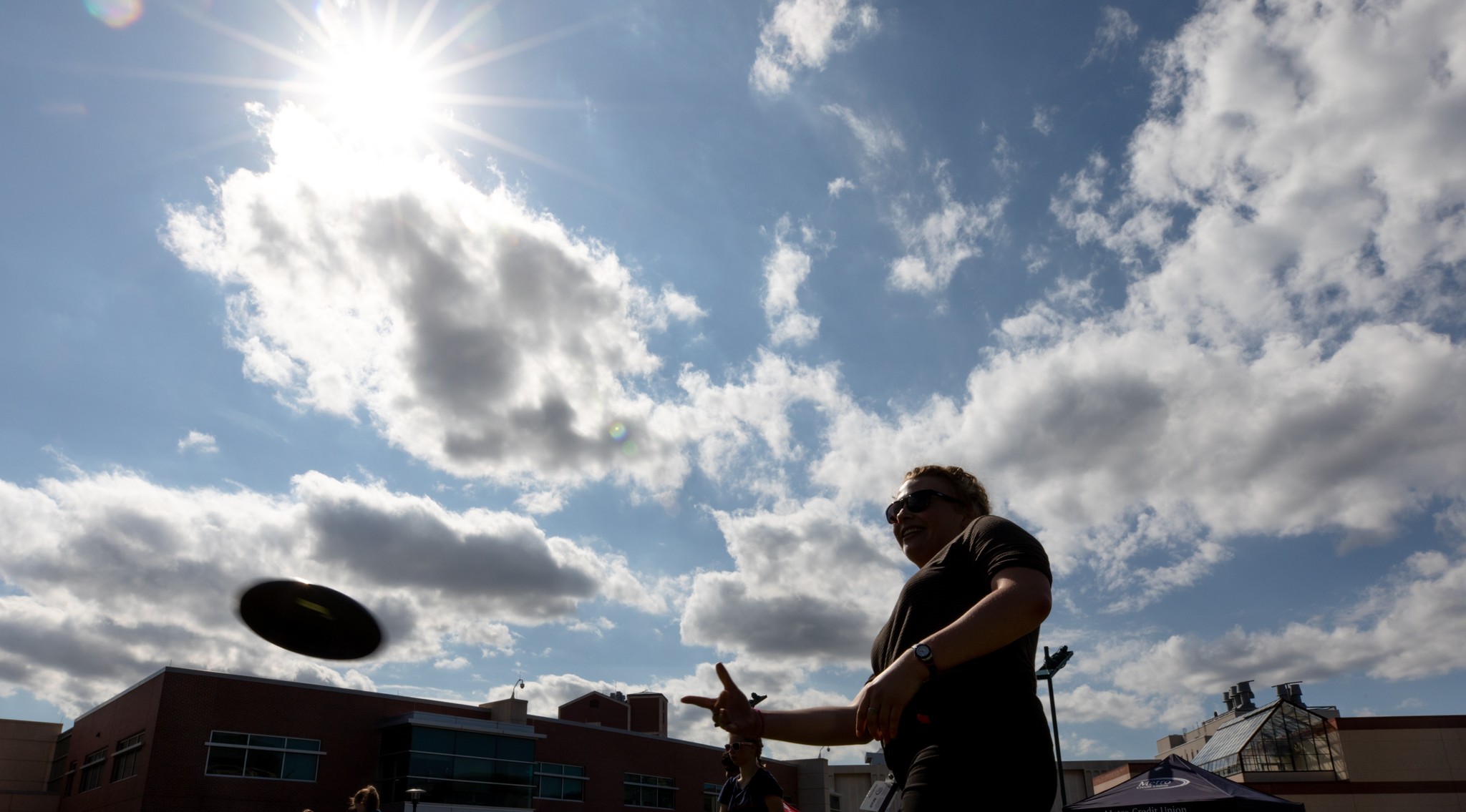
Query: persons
717,732,785,812
354,785,382,812
680,465,1058,812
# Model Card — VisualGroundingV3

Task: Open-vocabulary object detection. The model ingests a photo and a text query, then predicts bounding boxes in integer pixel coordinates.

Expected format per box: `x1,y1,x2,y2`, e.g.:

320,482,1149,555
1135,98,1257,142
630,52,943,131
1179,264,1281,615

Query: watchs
911,643,939,683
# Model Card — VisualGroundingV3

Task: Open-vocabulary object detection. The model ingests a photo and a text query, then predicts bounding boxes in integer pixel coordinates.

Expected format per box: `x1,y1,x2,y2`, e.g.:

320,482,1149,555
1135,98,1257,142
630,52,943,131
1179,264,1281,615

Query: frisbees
240,580,382,661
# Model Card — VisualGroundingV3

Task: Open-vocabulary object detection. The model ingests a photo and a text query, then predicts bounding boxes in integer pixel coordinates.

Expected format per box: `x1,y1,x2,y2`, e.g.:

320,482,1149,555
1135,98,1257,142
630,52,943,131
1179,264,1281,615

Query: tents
1062,754,1307,812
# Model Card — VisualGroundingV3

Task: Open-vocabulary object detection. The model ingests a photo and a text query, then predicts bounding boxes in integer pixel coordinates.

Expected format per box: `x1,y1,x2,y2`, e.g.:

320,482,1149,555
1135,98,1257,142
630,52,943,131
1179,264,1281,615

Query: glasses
886,489,967,524
725,742,755,753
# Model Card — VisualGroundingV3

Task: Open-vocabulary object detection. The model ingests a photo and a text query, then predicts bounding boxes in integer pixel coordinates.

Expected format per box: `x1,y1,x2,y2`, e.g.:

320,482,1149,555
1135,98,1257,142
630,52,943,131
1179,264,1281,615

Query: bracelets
750,708,763,740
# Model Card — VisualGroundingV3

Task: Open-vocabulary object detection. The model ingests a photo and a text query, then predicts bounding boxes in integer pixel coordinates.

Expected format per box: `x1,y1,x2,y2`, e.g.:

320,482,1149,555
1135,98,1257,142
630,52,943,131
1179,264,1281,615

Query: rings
868,708,878,712
